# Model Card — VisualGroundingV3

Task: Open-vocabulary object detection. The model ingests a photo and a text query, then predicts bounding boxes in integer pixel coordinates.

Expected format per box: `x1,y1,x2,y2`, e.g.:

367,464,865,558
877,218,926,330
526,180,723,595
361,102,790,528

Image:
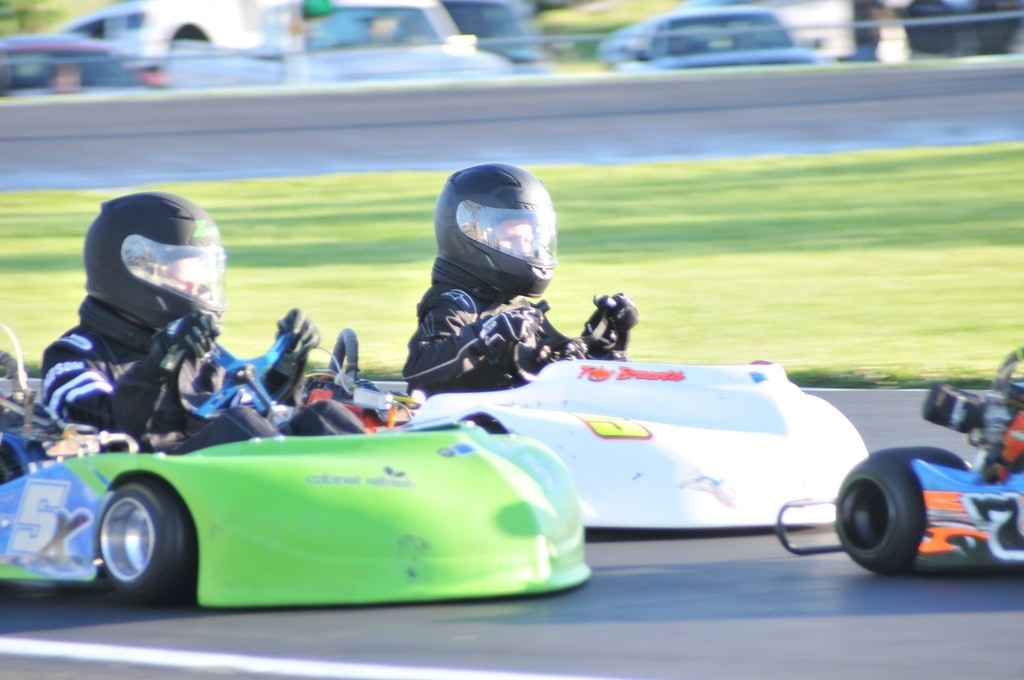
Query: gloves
277,307,320,351
479,306,544,345
161,310,222,358
595,294,641,330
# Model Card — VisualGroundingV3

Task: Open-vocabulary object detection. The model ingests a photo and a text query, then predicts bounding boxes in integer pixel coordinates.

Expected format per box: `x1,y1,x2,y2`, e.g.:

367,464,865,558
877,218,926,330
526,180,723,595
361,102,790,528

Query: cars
1,0,842,99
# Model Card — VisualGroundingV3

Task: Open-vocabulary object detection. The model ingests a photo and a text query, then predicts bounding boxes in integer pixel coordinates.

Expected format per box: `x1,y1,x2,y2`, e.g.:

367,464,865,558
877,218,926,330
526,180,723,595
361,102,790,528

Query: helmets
434,162,556,297
79,192,226,331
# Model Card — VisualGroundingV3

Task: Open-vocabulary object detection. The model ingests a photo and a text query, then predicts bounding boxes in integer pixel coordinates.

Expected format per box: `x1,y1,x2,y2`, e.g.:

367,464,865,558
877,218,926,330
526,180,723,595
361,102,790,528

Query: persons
402,162,639,394
37,192,320,453
